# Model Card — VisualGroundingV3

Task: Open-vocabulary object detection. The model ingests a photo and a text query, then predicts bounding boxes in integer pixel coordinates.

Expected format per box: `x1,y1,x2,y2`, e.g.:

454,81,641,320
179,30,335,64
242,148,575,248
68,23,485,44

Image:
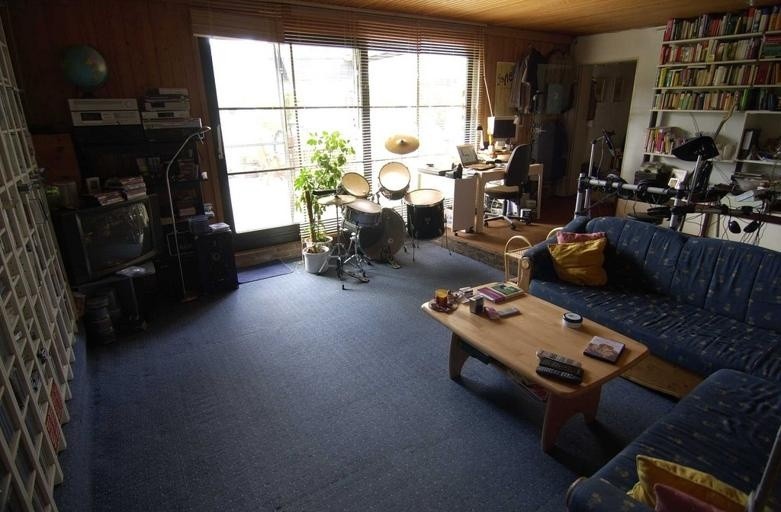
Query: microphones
601,128,617,156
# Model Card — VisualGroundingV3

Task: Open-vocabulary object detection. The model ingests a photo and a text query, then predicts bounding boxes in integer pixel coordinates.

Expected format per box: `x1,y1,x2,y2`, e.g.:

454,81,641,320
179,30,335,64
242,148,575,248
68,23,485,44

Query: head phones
728,205,761,234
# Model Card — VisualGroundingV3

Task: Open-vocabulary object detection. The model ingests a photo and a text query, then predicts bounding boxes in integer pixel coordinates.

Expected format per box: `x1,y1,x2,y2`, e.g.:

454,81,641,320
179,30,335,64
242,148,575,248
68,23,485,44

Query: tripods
319,206,366,290
343,224,374,278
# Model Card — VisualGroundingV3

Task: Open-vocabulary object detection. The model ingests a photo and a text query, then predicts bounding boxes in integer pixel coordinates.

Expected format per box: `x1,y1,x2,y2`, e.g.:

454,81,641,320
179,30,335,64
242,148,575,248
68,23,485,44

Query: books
83,147,200,205
582,334,625,364
504,366,550,402
729,169,781,201
477,280,527,304
642,3,781,161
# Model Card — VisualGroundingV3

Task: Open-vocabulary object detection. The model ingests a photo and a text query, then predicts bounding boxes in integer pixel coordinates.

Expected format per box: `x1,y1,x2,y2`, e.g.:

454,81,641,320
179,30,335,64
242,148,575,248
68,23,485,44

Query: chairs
482,143,533,230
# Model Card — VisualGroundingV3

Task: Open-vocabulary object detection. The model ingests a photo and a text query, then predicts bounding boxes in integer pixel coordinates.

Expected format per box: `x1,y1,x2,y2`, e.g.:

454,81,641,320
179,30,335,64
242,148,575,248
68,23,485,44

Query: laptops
457,143,496,171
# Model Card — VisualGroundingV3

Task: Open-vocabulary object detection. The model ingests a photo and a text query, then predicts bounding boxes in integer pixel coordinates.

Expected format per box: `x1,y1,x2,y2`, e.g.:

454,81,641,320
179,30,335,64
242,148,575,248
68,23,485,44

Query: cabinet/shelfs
28,117,207,344
611,28,781,249
0,18,81,511
416,166,476,237
502,225,563,284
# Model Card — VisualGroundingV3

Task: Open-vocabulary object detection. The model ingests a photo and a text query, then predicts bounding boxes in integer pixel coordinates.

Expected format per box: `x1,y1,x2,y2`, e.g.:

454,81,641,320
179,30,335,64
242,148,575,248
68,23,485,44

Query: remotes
539,356,584,377
536,365,582,385
536,348,582,368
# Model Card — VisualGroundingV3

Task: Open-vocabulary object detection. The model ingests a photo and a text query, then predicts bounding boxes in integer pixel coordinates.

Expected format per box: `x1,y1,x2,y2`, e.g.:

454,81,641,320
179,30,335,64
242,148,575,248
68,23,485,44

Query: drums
341,198,381,227
377,161,410,200
404,188,444,239
337,172,370,198
359,209,405,259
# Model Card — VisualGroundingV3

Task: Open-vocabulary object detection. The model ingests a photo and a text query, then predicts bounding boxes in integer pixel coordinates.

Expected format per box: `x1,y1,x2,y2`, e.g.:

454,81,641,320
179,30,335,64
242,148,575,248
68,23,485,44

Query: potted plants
292,127,356,275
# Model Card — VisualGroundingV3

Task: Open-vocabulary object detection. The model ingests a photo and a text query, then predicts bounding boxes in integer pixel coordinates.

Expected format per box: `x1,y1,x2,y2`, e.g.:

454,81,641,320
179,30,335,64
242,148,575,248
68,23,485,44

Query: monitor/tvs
72,196,158,280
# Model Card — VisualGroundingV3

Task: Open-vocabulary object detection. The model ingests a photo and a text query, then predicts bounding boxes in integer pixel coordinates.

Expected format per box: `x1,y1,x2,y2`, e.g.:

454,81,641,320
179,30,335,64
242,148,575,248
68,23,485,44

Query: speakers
198,230,239,292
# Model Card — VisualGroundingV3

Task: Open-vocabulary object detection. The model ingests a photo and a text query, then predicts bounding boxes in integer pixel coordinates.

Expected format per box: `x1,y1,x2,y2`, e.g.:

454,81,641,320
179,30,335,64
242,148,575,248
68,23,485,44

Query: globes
58,46,107,98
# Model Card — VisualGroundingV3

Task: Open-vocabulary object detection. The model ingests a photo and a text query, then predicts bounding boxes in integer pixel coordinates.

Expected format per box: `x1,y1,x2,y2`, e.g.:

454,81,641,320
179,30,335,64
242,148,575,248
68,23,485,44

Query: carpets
236,258,294,284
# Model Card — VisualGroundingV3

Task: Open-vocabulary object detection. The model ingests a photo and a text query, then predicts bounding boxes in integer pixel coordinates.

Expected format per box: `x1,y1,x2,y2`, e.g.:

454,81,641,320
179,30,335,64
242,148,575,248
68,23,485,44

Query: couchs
515,214,780,512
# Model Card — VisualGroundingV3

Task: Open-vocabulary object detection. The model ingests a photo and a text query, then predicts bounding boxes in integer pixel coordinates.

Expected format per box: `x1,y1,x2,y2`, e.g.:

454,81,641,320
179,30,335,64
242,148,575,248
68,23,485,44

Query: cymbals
385,135,420,153
319,195,357,205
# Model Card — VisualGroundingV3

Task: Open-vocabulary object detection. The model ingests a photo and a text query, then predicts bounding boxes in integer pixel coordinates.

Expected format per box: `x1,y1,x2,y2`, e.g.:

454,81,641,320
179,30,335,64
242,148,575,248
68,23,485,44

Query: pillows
627,454,751,512
546,238,608,290
556,232,607,245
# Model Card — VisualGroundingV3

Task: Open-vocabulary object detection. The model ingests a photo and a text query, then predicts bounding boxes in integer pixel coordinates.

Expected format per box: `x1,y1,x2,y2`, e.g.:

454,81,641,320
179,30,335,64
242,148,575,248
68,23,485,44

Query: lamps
165,124,212,304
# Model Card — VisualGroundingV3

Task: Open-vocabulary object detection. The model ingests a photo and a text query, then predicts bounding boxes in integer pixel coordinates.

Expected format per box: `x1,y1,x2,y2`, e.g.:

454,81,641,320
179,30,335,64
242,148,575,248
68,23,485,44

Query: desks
448,159,544,233
